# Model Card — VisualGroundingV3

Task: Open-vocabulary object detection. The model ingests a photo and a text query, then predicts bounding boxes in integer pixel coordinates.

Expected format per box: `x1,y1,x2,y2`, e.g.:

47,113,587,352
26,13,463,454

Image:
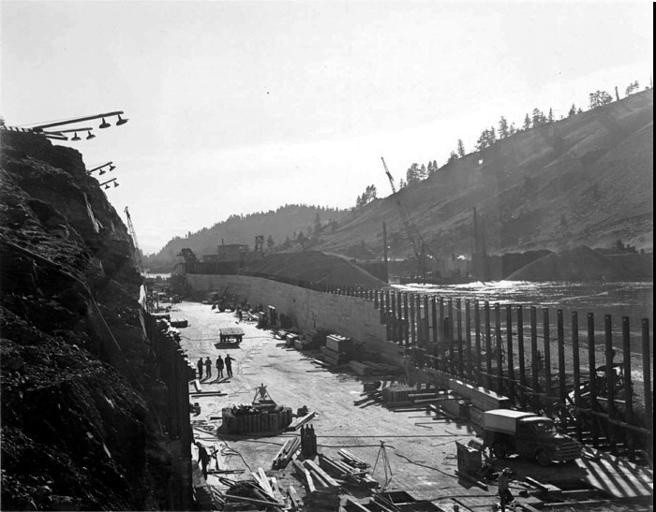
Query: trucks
483,409,582,467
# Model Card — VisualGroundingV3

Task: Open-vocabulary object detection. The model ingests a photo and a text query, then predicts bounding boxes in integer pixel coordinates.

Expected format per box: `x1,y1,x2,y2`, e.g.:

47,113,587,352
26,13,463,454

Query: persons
216,355,224,378
197,358,206,379
498,467,514,512
196,442,210,480
225,354,236,378
205,357,212,378
